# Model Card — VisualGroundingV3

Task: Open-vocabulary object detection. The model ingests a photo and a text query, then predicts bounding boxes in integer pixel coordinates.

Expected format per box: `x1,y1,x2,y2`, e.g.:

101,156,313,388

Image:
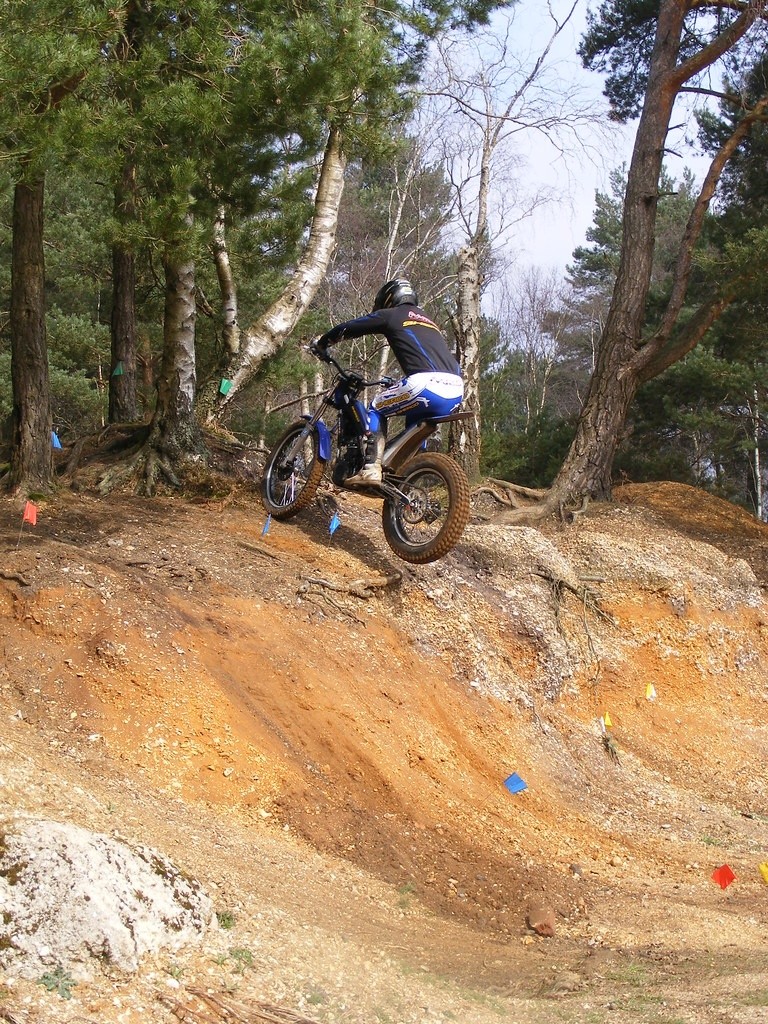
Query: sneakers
344,464,383,486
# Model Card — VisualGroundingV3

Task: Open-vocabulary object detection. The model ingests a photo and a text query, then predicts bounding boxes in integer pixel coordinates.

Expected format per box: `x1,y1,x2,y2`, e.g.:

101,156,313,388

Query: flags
329,513,340,534
111,362,123,377
220,378,233,396
24,501,36,526
52,433,61,448
503,772,527,794
711,865,736,890
262,516,270,536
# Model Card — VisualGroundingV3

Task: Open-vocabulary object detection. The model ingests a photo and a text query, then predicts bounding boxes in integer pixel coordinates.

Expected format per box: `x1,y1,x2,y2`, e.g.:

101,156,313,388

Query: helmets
375,279,418,309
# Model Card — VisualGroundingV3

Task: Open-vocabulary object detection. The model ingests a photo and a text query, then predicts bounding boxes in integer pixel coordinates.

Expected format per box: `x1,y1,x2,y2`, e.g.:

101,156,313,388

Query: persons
310,279,464,485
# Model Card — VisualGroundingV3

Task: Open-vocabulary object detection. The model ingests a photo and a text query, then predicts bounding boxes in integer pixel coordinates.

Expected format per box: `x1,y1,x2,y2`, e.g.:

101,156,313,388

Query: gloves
311,341,326,361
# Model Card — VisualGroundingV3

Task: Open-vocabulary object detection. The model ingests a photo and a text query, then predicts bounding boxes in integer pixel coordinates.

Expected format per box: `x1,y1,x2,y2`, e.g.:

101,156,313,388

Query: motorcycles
259,344,476,565
